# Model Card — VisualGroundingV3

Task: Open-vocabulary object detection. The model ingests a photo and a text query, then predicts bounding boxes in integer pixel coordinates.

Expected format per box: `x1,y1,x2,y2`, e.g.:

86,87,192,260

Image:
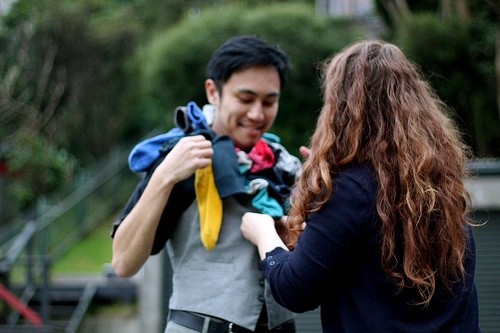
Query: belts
166,310,297,333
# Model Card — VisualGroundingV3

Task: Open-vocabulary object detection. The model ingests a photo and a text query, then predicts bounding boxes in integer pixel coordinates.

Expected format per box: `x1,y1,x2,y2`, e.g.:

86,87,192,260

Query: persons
111,35,302,333
240,38,490,333
